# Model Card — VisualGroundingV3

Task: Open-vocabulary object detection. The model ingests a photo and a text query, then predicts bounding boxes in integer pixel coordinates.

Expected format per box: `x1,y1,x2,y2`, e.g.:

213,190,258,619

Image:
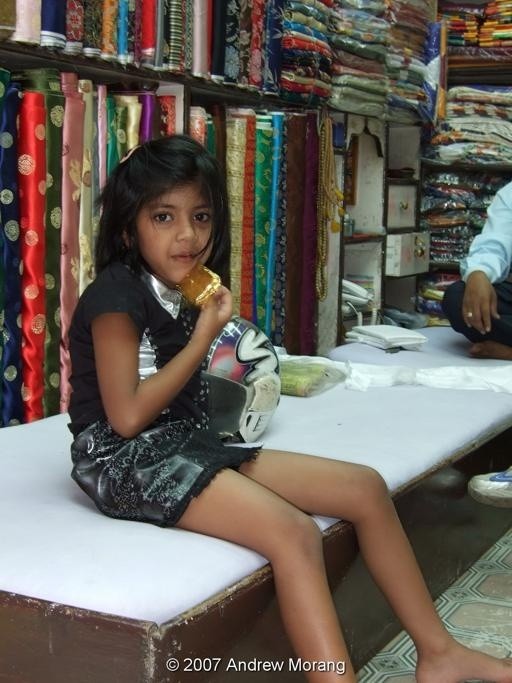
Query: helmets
203,315,282,444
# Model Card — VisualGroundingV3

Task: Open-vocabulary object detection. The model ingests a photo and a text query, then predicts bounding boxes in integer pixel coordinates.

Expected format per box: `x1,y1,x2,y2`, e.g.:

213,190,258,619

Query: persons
439,177,511,362
65,131,510,682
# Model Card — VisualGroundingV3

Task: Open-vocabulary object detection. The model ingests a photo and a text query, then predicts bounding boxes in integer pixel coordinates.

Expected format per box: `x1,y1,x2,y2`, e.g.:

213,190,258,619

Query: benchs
0,351,512,681
322,322,511,373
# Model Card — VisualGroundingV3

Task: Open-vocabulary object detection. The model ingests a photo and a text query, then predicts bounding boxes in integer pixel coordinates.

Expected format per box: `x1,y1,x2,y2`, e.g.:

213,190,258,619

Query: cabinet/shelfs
418,1,512,323
326,1,442,335
0,0,326,426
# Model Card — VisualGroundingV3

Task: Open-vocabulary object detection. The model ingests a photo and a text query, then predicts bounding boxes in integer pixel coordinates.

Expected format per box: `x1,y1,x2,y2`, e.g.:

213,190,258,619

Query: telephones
341,278,374,305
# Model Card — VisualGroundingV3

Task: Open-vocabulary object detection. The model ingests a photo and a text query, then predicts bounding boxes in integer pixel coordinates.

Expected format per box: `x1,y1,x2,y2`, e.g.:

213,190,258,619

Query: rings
464,311,472,317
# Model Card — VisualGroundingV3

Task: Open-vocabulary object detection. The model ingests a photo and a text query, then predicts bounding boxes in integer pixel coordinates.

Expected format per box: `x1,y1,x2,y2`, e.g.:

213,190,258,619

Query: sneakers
469,466,512,511
